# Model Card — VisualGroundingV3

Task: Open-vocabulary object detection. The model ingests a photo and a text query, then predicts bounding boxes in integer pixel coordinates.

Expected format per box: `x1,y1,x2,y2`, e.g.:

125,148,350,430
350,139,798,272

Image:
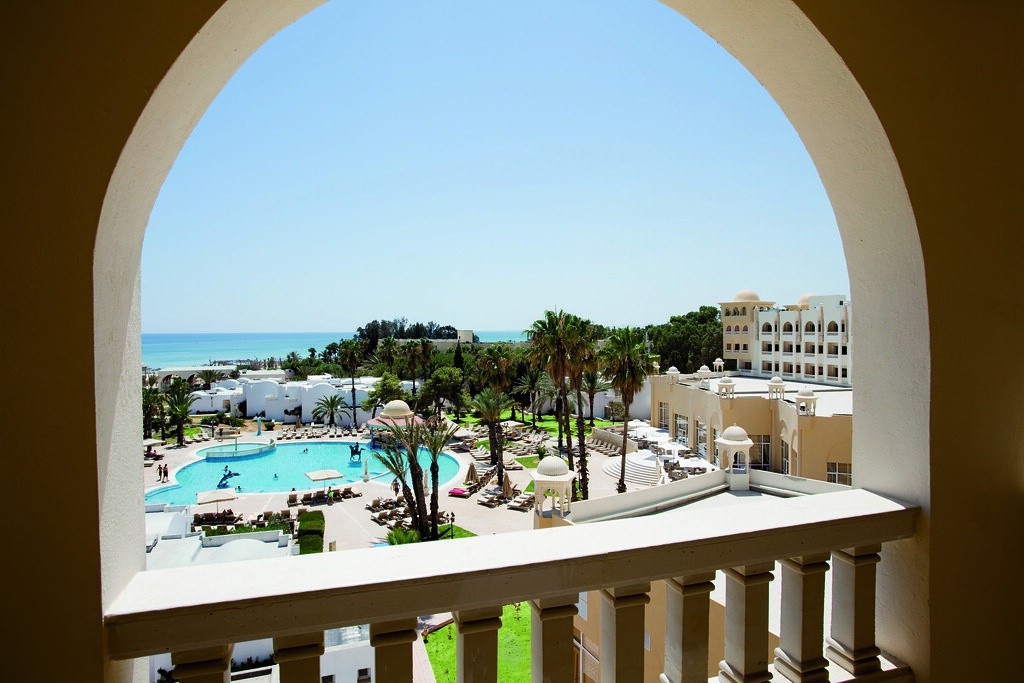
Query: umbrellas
496,420,525,431
676,417,688,425
502,471,513,504
464,462,480,485
196,488,238,514
143,438,166,446
623,419,649,436
305,469,343,495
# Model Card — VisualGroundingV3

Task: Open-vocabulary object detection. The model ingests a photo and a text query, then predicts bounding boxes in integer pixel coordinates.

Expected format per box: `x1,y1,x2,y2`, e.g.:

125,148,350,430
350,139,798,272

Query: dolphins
217,470,241,486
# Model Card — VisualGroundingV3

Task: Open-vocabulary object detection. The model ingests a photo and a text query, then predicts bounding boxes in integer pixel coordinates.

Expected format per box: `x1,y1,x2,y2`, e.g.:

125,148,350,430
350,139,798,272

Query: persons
144,449,157,458
163,464,170,483
156,464,164,481
393,479,401,497
503,422,523,438
327,486,335,503
223,448,309,491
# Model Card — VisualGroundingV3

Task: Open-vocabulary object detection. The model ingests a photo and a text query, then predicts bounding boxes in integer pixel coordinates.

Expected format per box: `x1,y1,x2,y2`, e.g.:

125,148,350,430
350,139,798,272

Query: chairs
277,423,367,439
184,436,193,444
449,424,621,512
193,434,202,442
144,449,165,467
366,495,445,530
287,487,362,506
202,433,211,441
191,508,307,532
631,437,707,482
